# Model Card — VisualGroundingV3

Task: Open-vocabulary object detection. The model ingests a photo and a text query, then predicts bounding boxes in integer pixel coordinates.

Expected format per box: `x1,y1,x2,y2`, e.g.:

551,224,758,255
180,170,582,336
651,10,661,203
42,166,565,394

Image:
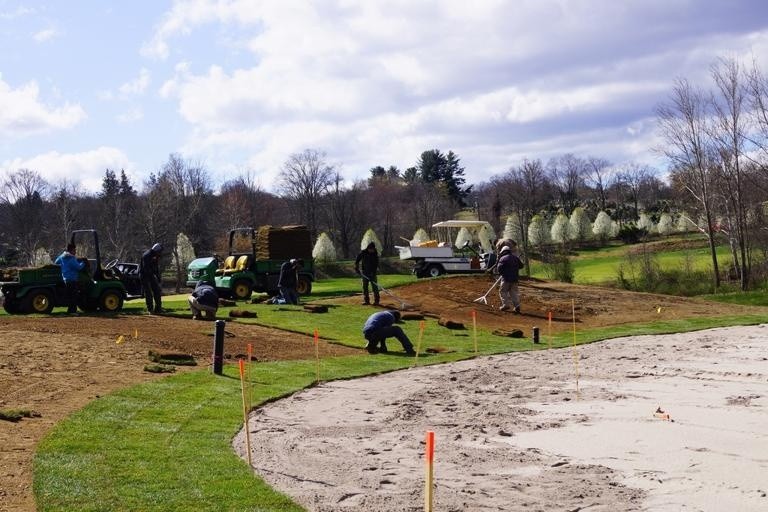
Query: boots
361,297,380,306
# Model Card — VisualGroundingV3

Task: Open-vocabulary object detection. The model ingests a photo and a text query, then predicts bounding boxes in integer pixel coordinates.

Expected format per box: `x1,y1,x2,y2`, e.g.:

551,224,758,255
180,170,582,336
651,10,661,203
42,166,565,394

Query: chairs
223,255,248,275
216,256,235,276
452,244,464,258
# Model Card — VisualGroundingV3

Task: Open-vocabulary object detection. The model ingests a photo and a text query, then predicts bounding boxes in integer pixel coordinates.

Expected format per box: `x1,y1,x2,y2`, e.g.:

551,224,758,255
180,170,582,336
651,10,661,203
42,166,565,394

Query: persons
138,242,170,315
353,241,382,307
265,254,301,307
188,279,218,321
54,243,88,317
497,245,525,313
362,309,418,356
494,237,517,275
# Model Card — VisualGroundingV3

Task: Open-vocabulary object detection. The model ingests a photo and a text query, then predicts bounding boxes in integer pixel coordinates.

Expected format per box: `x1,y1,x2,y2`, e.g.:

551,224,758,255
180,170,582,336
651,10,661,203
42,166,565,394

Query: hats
152,243,164,253
390,311,400,320
367,242,376,250
499,246,510,254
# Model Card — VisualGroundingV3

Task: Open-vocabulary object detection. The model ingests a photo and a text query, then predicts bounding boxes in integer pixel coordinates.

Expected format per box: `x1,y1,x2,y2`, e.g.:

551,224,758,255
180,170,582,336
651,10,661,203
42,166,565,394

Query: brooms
361,273,418,310
473,277,500,306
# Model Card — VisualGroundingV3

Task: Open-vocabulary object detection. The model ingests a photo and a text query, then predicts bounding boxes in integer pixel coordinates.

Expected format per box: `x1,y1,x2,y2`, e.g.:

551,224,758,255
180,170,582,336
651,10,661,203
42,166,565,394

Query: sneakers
500,304,520,314
193,315,199,320
70,312,80,317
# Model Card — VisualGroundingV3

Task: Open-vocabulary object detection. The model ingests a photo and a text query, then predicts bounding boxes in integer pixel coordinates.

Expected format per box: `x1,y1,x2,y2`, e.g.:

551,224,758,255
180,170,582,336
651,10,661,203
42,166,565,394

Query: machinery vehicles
186,228,315,299
0,229,144,315
398,216,501,279
